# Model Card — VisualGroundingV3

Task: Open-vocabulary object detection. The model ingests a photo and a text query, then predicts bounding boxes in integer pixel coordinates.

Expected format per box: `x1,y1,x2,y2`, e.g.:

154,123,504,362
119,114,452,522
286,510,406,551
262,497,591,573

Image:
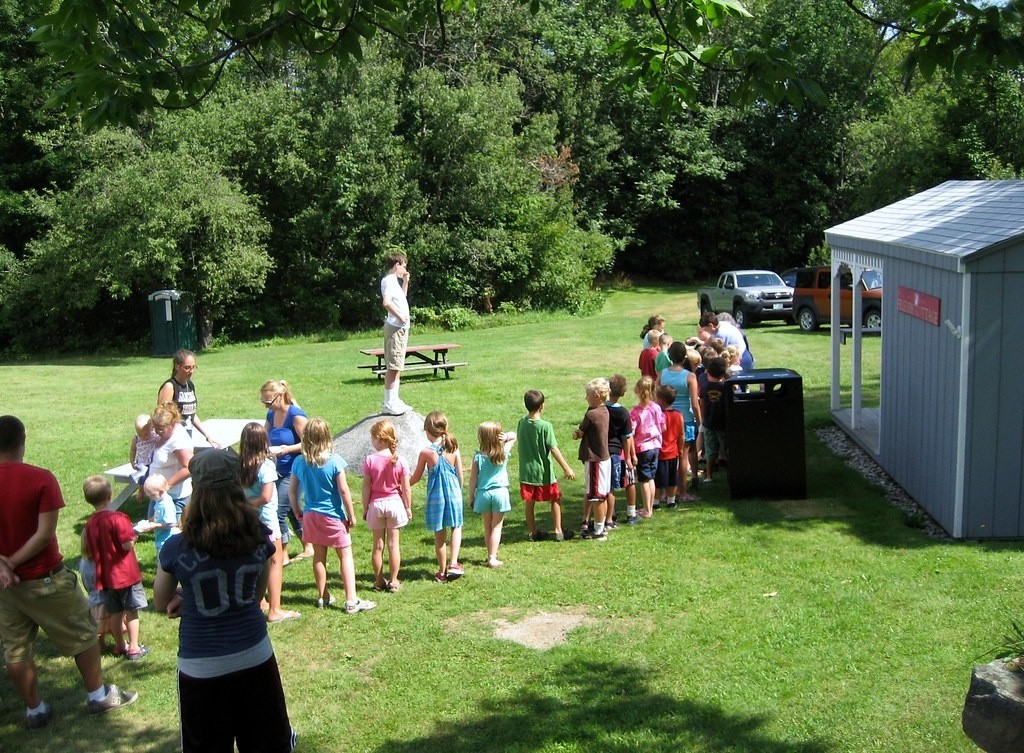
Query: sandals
435,571,448,583
373,578,389,591
128,643,150,659
113,641,130,656
387,579,401,592
318,594,335,608
447,563,464,575
345,598,377,613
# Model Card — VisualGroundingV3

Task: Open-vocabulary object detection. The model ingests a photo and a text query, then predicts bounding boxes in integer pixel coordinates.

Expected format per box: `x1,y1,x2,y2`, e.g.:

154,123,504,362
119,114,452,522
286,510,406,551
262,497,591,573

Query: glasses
179,364,198,371
260,393,280,407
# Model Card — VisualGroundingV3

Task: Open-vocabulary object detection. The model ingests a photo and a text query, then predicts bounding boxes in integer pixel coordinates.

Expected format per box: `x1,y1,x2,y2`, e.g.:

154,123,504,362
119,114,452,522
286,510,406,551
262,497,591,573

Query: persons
362,420,412,592
157,348,222,449
580,374,634,531
148,401,195,522
468,421,517,567
409,411,464,583
287,418,377,614
83,476,149,660
75,527,127,653
0,415,138,729
517,389,576,542
260,380,314,565
129,415,161,504
381,251,414,415
154,447,298,753
133,473,182,560
572,377,611,542
238,422,302,624
621,311,756,518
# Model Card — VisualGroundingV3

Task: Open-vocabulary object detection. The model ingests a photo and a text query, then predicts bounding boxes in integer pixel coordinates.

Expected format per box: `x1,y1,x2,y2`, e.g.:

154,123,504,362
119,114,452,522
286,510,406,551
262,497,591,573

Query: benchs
357,359,450,368
372,362,469,373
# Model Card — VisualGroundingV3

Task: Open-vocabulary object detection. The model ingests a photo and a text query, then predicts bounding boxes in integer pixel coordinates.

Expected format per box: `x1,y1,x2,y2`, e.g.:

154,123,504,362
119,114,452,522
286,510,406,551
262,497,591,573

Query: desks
192,419,266,456
360,344,461,380
104,463,139,511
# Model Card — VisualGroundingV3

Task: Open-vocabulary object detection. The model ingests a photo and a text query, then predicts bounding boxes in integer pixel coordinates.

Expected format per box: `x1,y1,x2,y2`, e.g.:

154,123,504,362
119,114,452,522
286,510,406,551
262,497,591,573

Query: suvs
791,264,882,337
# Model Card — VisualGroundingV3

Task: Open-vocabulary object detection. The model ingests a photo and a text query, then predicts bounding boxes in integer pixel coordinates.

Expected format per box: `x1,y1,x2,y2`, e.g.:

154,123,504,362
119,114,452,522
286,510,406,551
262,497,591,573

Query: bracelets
206,437,210,441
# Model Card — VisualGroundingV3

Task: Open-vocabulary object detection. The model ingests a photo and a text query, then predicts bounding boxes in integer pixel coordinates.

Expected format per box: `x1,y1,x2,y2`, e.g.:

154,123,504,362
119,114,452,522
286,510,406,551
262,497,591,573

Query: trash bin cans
148,289,197,358
724,368,808,502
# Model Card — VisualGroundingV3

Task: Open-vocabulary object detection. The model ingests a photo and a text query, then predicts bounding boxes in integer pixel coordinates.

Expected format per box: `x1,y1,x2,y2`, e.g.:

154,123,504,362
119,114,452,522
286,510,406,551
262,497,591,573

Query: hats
188,448,239,488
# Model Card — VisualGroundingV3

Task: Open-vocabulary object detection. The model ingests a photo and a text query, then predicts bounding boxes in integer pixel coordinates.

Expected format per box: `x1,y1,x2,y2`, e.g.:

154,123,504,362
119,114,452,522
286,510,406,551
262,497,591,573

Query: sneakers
27,702,52,732
88,684,138,717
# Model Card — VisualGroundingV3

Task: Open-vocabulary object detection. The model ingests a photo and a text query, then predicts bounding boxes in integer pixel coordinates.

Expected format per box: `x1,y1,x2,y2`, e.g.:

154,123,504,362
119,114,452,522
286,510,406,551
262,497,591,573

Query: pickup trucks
697,269,798,329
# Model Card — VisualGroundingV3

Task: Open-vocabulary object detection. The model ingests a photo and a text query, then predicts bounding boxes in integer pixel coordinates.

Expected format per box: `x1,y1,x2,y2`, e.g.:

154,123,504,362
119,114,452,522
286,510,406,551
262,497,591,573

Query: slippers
272,609,301,623
261,602,269,614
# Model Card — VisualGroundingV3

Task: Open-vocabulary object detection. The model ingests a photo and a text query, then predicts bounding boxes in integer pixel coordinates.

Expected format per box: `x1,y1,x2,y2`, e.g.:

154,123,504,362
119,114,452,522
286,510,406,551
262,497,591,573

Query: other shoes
529,531,549,541
481,561,489,566
399,399,414,411
581,455,727,542
554,530,574,541
489,560,503,567
382,401,407,416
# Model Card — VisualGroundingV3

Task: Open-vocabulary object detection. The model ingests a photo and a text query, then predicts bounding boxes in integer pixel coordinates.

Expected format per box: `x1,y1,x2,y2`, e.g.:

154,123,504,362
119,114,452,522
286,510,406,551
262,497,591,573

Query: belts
37,561,64,580
